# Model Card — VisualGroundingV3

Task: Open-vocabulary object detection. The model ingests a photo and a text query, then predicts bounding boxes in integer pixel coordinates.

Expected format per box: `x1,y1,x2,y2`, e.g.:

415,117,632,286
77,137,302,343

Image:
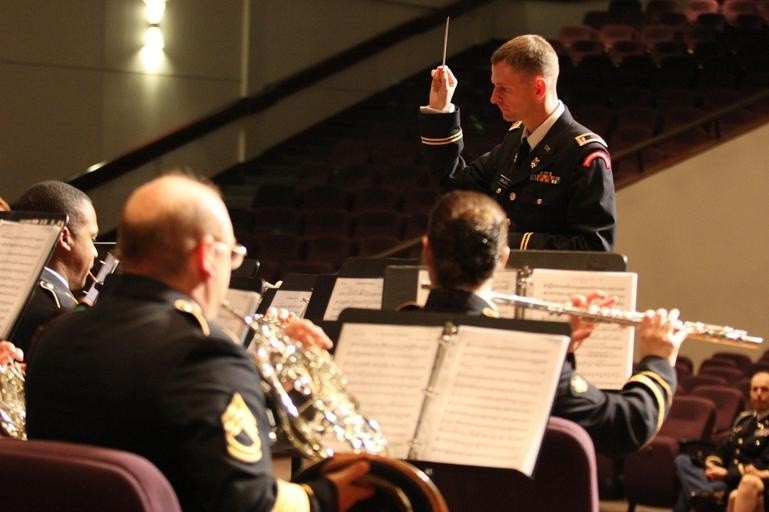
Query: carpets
213,244,247,271
510,137,530,175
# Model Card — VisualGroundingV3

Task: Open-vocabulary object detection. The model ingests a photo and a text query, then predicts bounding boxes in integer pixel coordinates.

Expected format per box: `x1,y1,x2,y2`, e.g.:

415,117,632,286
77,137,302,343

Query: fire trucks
421,281,765,351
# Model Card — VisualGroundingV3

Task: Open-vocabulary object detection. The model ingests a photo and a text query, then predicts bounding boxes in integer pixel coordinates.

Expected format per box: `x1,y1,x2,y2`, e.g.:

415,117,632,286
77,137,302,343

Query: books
416,267,637,389
218,289,260,343
309,320,572,476
0,220,60,339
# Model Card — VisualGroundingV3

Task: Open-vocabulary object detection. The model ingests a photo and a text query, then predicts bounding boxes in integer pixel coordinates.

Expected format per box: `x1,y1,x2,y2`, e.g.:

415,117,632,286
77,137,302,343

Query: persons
416,34,617,253
674,372,769,512
24,172,375,512
402,192,692,455
10,182,98,353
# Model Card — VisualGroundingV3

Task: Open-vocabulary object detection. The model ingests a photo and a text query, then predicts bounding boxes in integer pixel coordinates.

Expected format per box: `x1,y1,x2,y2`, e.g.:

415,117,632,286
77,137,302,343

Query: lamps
600,349,769,512
404,415,600,511
0,434,184,512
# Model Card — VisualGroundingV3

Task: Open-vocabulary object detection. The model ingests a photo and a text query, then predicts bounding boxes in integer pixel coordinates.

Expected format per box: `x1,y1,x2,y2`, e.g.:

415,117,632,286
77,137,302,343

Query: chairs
404,415,600,511
231,0,767,275
0,434,184,512
600,349,769,512
